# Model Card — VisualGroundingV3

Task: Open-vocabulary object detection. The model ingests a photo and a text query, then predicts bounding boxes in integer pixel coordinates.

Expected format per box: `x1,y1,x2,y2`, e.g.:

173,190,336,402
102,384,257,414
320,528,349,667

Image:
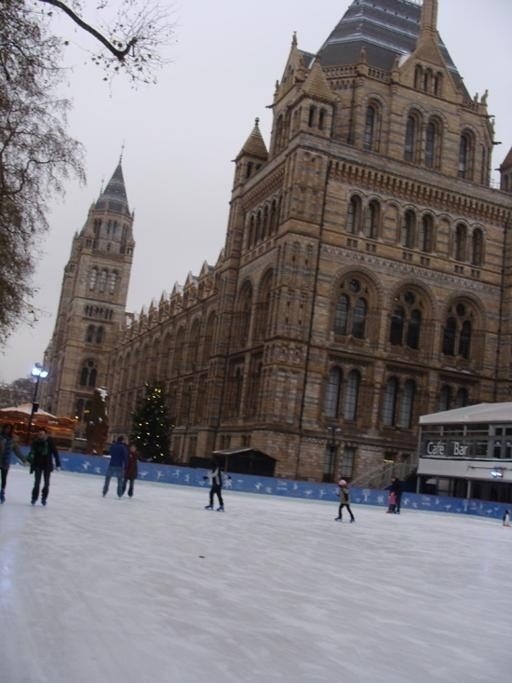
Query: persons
26,427,61,506
335,479,354,523
0,423,30,503
503,509,512,528
203,463,231,512
384,475,402,514
103,435,138,497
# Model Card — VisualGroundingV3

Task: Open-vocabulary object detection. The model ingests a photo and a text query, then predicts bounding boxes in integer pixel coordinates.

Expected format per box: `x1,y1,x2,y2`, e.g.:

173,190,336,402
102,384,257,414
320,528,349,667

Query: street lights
25,362,49,444
326,423,342,483
